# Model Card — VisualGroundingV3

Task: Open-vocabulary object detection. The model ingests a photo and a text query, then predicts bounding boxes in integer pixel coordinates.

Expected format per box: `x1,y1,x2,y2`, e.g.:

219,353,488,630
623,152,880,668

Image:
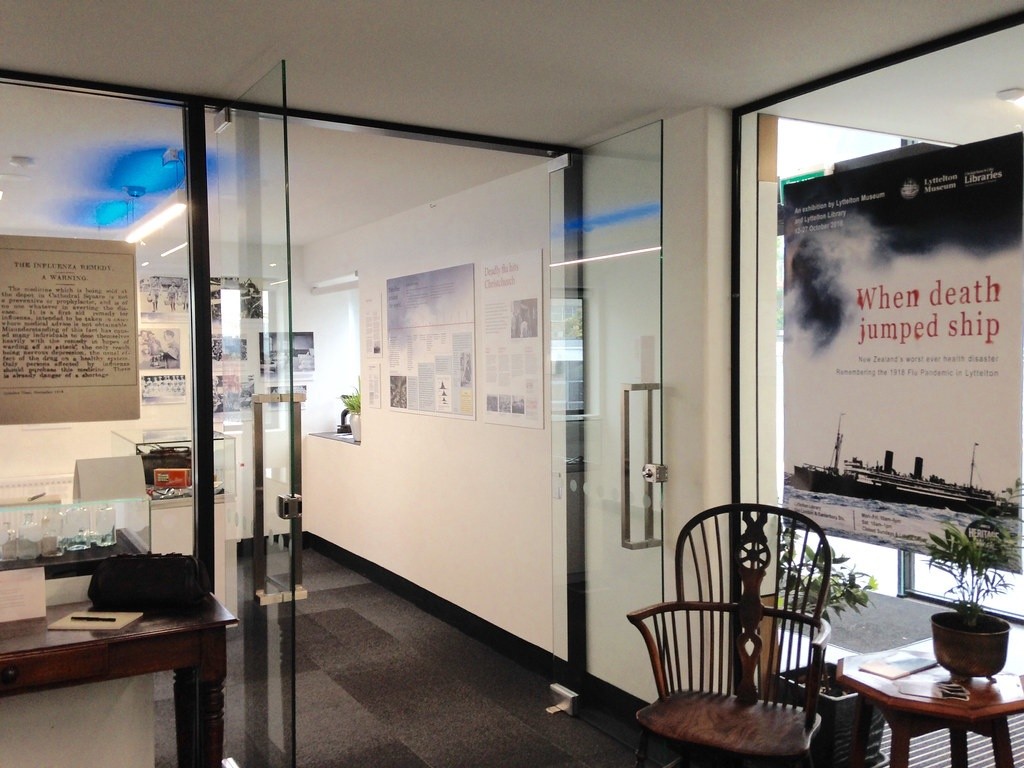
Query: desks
0,592,240,768
837,649,1024,768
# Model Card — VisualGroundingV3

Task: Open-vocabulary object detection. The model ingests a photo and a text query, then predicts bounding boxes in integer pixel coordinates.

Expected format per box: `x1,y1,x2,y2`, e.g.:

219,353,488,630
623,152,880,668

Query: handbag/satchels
87,550,211,616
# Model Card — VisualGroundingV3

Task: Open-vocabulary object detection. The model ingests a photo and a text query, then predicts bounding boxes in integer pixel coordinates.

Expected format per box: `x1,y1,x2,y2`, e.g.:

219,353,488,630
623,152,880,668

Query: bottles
41,506,64,557
96,504,117,547
18,513,40,559
66,498,91,551
0,521,17,561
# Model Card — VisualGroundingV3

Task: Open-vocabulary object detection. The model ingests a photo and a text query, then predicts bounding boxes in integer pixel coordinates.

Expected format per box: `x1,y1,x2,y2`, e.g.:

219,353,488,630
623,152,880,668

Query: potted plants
902,478,1024,677
339,387,361,442
765,528,890,768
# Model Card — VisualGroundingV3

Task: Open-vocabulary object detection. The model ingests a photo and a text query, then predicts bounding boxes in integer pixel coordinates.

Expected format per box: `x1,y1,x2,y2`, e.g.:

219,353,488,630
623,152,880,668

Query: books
859,651,938,680
47,612,143,629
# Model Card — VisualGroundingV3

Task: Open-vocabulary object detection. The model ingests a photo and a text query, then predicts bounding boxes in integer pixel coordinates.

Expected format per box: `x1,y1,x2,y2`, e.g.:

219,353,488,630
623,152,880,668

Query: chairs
629,503,831,768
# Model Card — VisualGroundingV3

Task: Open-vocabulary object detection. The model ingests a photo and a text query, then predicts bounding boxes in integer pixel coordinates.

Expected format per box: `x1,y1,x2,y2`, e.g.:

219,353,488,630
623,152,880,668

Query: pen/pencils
71,615,116,622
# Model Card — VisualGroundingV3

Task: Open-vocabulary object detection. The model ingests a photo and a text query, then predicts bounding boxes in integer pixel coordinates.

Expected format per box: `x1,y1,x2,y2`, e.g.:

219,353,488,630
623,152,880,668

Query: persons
147,277,161,313
167,279,188,312
139,330,178,368
141,375,186,395
